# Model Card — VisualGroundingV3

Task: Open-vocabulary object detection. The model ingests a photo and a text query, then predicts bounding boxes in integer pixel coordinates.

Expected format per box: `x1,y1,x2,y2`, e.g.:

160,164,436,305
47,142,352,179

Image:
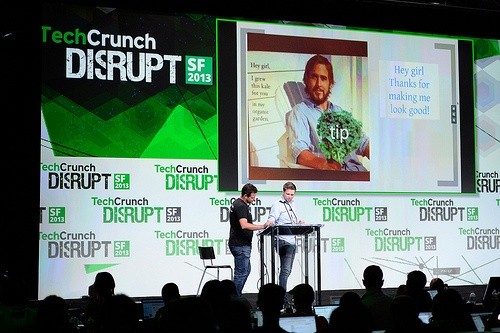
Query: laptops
279,314,317,333
418,311,434,325
329,296,342,305
250,309,263,327
426,289,439,301
481,277,500,305
313,304,340,324
142,298,165,321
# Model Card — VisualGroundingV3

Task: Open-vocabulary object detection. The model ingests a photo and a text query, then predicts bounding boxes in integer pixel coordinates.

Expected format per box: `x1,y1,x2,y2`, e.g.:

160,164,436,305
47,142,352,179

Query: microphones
279,200,293,226
284,201,298,224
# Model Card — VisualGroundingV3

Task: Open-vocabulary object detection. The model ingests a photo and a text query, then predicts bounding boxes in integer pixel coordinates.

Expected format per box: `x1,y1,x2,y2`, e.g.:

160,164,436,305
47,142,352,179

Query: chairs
197,247,233,296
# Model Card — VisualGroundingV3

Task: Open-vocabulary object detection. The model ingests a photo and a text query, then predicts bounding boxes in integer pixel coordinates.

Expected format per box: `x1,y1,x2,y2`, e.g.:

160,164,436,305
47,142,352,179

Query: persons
228,183,273,296
266,182,304,292
0,265,500,333
287,54,370,171
